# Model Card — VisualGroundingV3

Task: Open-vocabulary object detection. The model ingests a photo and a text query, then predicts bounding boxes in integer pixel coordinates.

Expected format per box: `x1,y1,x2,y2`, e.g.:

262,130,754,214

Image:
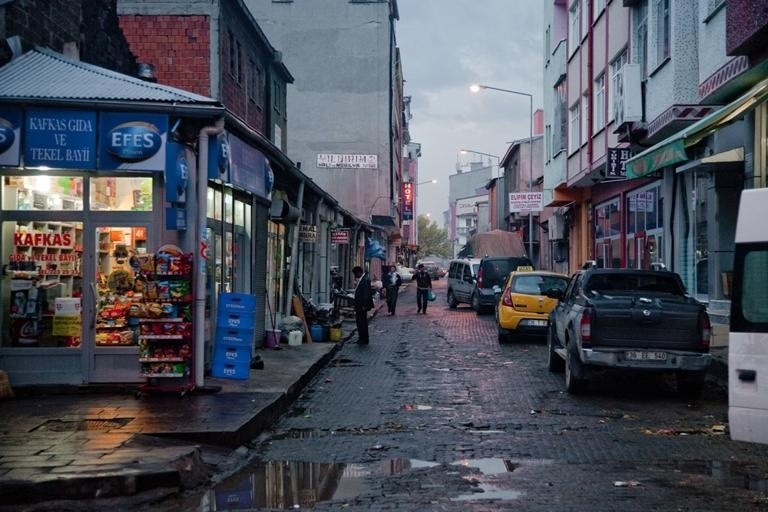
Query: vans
446,258,482,308
726,187,767,446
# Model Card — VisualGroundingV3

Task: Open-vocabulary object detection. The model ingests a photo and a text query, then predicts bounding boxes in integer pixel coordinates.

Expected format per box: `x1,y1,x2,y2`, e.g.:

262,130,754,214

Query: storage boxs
210,292,258,381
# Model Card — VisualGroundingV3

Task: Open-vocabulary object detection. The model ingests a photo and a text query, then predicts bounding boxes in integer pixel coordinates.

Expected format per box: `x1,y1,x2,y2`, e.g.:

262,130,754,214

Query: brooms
265,290,283,350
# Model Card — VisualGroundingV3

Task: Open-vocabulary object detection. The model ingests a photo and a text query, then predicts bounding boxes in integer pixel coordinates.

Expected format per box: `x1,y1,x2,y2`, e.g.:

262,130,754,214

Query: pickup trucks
545,260,713,400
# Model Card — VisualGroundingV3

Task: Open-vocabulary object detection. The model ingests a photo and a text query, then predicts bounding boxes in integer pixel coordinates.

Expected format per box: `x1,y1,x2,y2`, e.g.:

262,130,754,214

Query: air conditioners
609,61,643,132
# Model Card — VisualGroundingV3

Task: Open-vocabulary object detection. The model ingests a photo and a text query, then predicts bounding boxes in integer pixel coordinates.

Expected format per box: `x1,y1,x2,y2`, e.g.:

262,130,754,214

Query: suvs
471,255,537,312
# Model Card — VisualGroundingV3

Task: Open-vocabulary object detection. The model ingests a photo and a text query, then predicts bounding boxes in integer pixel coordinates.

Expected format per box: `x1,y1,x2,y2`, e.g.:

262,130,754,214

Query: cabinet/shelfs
133,267,196,399
4,185,111,276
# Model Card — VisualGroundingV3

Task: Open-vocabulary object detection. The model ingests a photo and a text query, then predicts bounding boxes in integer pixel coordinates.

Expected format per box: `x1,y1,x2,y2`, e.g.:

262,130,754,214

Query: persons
382,264,402,316
331,270,344,305
412,264,433,314
352,266,375,346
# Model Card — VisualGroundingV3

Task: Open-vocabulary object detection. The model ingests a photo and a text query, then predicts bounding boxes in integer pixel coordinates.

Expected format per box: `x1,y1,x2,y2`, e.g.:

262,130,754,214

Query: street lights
414,179,438,245
459,149,501,228
468,83,533,263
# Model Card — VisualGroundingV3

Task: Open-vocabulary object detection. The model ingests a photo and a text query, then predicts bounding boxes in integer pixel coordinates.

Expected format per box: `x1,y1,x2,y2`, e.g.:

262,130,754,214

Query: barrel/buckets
288,330,302,346
266,330,282,348
331,328,342,341
311,324,323,342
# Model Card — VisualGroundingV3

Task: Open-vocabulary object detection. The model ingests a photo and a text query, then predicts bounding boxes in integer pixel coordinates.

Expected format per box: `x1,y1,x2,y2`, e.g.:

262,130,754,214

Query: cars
397,259,446,283
494,269,569,342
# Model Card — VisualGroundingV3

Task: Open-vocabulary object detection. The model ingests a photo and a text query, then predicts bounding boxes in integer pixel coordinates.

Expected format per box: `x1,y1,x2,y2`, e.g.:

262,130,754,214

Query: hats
419,265,424,268
